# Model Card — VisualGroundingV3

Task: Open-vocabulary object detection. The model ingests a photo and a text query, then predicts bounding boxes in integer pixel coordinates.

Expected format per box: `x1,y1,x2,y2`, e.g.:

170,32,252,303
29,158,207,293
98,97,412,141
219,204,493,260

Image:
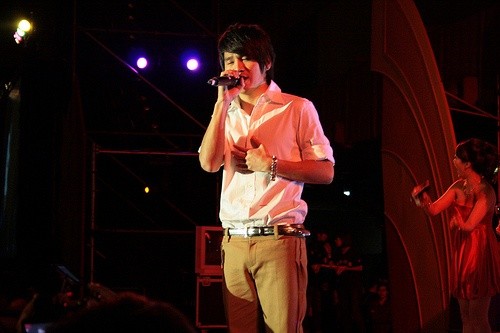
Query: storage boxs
194,226,227,328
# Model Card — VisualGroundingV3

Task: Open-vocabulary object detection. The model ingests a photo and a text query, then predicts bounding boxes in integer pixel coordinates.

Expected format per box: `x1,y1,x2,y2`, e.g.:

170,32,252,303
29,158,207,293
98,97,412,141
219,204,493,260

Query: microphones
207,75,243,87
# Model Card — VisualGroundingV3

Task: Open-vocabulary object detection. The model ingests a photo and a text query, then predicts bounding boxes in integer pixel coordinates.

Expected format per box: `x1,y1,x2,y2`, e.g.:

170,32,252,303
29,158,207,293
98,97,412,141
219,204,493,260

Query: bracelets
271,156,276,181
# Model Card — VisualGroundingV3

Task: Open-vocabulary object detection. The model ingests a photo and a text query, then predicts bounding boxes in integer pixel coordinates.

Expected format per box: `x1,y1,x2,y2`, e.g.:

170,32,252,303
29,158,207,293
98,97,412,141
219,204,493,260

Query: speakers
195,277,227,329
195,226,225,276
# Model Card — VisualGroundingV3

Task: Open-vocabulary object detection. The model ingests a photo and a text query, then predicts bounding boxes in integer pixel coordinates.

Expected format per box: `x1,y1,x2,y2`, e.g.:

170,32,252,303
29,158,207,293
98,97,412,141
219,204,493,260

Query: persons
411,139,500,333
200,26,335,333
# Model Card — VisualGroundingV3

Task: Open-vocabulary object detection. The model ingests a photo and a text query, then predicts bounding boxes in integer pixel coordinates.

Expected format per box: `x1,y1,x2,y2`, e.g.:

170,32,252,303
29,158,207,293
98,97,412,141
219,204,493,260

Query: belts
222,227,312,237
